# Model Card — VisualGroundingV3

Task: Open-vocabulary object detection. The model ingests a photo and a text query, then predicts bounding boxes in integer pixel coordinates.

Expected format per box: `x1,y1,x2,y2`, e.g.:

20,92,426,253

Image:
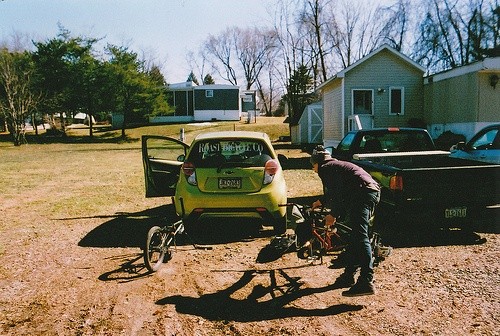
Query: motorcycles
257,201,390,263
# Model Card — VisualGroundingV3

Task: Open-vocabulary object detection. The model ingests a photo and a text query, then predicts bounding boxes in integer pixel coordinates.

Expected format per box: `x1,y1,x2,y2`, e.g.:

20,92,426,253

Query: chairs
365,139,383,151
400,139,422,150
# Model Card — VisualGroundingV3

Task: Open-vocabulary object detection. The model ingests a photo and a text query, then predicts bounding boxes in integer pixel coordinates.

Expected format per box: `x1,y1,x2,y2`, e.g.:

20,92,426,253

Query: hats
310,145,331,164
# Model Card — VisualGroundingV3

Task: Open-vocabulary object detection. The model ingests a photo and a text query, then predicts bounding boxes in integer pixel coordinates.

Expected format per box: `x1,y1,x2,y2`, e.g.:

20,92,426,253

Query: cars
141,130,287,236
449,122,500,164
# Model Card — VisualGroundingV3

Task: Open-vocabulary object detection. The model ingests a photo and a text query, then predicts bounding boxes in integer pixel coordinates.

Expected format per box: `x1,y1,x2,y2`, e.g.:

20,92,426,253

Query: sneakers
334,271,355,287
343,276,374,296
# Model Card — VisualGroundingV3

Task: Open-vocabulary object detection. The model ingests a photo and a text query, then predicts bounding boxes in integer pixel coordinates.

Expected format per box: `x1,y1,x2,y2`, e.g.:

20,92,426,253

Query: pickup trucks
324,127,500,219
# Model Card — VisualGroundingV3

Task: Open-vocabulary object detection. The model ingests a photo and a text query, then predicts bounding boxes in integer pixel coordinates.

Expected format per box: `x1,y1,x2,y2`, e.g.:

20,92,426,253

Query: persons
310,144,381,297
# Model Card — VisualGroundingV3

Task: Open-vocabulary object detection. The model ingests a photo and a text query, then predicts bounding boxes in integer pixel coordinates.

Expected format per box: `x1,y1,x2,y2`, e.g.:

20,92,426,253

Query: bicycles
141,219,187,271
